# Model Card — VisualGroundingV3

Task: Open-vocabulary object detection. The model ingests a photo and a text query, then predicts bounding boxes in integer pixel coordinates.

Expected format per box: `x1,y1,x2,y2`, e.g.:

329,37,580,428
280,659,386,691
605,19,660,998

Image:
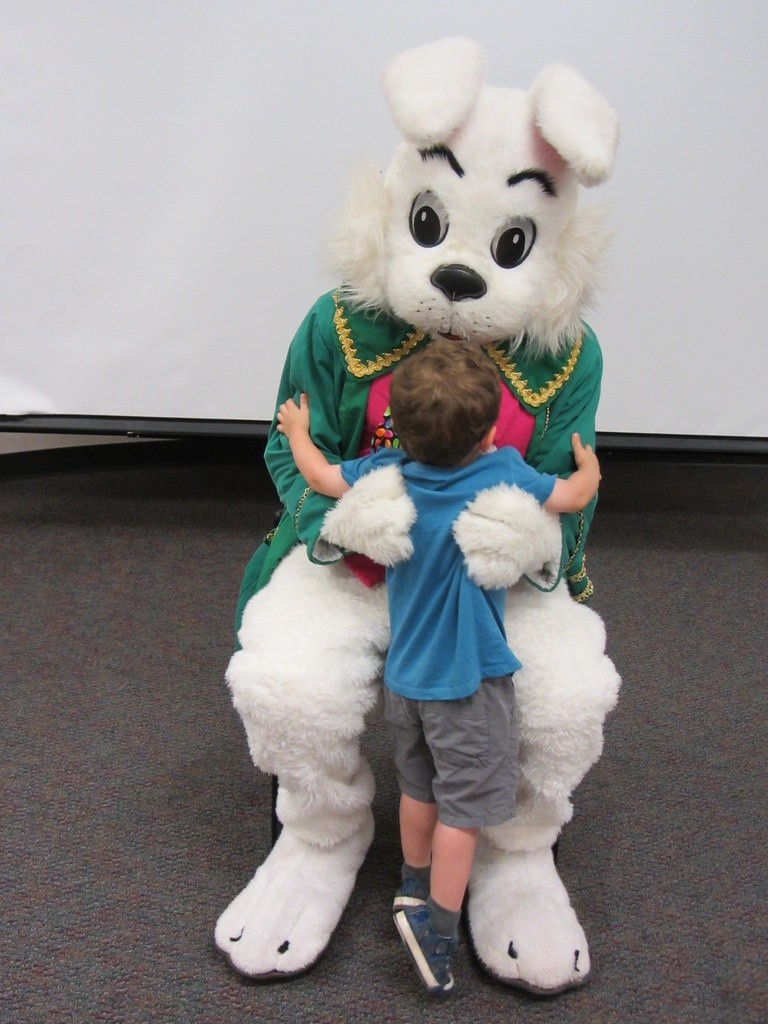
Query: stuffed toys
213,38,620,995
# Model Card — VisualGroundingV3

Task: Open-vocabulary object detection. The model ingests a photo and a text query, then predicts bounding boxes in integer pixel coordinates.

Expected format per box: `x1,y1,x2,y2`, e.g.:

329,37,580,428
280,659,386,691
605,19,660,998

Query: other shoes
393,878,431,913
393,904,455,996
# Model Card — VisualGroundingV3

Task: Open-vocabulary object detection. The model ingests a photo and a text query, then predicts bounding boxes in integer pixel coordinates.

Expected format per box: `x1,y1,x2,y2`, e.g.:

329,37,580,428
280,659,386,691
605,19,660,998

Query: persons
276,341,602,995
